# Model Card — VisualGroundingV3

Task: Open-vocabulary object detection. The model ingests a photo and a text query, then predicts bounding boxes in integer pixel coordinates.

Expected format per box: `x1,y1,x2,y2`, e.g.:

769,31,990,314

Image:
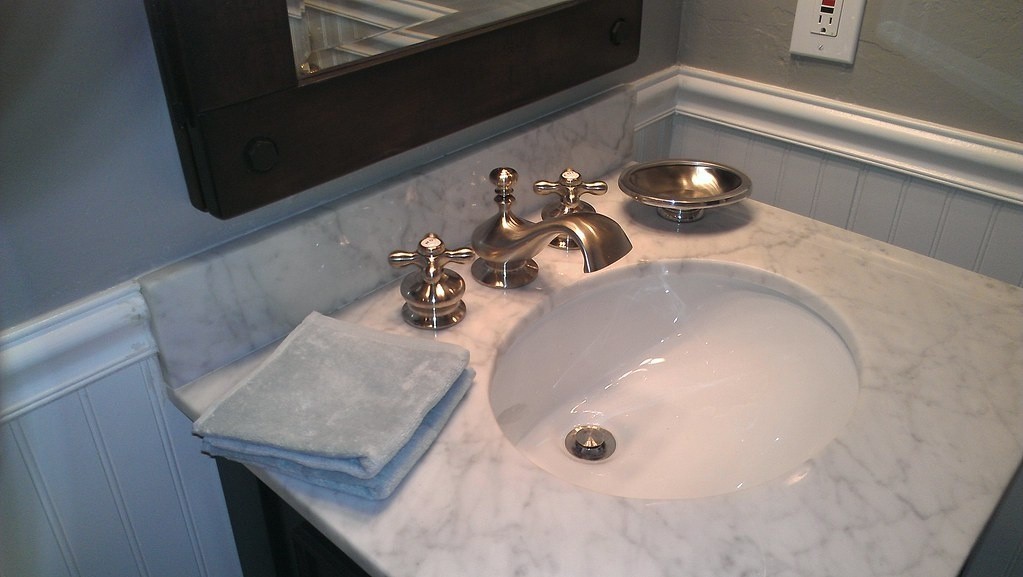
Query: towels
185,310,476,502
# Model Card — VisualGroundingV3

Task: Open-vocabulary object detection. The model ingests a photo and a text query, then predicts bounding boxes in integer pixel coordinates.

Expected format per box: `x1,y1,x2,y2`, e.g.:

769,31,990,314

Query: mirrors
139,0,646,221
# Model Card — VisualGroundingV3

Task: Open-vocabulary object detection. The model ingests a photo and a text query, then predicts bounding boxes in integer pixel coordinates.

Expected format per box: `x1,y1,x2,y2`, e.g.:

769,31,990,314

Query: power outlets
787,2,870,66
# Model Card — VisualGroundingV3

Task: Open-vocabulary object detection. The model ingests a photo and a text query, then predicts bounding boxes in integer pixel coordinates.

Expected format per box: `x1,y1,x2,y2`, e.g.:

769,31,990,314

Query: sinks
487,258,865,501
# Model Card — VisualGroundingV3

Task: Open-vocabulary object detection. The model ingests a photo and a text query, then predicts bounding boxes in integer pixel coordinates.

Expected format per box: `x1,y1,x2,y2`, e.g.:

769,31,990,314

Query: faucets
470,166,633,290
